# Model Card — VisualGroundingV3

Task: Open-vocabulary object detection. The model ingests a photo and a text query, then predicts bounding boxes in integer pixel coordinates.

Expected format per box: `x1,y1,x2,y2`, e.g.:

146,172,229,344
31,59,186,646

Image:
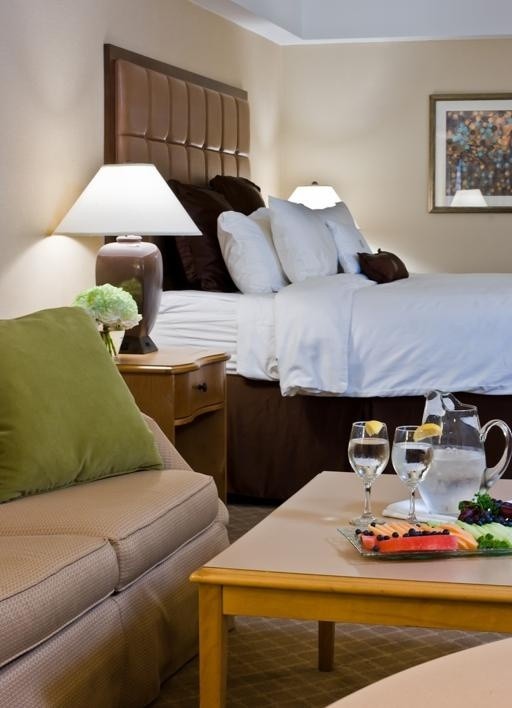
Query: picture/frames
429,93,511,214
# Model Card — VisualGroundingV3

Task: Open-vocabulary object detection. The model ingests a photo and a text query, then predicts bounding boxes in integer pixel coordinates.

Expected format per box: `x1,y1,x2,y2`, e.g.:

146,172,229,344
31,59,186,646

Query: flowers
74,284,143,355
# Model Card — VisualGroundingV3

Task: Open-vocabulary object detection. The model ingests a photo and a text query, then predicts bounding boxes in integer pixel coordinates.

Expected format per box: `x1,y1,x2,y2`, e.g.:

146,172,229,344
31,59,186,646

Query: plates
336,522,512,560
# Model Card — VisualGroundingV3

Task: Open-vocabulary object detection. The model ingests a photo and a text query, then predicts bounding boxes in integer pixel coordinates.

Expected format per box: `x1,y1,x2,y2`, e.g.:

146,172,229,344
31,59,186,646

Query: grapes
458,492,512,527
356,521,449,552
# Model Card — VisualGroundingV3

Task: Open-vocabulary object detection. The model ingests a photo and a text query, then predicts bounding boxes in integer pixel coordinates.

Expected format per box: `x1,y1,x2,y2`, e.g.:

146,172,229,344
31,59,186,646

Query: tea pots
415,388,512,517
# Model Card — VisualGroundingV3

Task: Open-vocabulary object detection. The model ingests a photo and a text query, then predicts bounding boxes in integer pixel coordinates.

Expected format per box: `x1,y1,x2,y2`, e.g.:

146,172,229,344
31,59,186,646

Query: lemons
366,420,382,437
412,423,443,441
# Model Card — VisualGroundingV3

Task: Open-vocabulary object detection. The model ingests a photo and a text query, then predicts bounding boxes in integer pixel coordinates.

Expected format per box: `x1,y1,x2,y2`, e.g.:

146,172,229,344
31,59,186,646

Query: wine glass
348,419,391,527
392,422,434,524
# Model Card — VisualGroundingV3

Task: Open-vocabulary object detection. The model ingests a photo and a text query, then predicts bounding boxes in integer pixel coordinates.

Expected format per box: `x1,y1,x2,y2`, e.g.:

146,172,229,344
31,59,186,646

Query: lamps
51,162,204,354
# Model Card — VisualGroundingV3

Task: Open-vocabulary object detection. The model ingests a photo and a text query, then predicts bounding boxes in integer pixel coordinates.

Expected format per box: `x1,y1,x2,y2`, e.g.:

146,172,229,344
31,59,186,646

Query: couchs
0,308,231,708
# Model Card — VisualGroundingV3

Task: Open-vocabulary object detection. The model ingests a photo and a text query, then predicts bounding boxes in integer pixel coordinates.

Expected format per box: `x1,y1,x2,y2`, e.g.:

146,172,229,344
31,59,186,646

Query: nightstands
107,348,232,516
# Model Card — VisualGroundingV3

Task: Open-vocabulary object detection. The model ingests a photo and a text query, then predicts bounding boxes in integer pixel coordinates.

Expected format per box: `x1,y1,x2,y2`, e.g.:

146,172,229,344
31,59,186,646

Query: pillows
153,172,413,297
0,305,164,504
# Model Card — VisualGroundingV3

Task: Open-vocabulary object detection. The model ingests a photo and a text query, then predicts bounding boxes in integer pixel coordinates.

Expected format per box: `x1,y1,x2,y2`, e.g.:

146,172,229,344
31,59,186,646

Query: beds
101,44,511,506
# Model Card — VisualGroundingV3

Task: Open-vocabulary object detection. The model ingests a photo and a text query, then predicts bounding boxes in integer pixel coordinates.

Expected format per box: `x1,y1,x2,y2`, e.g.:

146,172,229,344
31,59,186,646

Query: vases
102,329,126,357
